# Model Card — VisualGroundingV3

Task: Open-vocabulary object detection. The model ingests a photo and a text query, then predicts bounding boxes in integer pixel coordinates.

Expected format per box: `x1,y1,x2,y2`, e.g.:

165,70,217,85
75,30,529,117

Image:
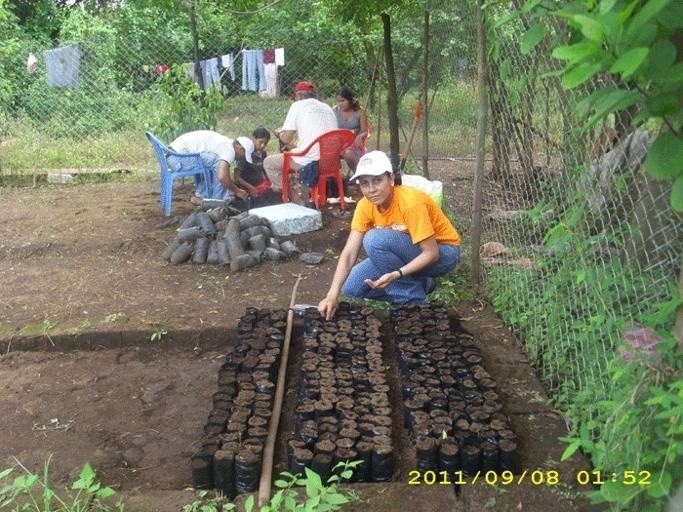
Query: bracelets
394,268,403,280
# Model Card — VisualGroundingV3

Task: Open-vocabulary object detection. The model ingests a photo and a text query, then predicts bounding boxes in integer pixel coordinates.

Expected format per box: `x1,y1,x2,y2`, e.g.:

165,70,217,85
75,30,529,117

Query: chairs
145,131,210,217
282,129,356,210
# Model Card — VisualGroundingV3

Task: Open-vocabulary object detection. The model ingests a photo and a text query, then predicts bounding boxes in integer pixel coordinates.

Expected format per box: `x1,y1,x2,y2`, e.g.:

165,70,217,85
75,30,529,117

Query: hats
347,150,394,182
291,80,316,93
236,136,254,164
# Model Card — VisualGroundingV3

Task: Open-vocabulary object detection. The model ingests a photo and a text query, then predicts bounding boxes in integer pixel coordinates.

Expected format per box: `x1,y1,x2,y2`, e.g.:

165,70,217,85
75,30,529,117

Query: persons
317,149,461,322
262,81,338,208
332,87,369,201
166,130,255,205
233,126,274,209
